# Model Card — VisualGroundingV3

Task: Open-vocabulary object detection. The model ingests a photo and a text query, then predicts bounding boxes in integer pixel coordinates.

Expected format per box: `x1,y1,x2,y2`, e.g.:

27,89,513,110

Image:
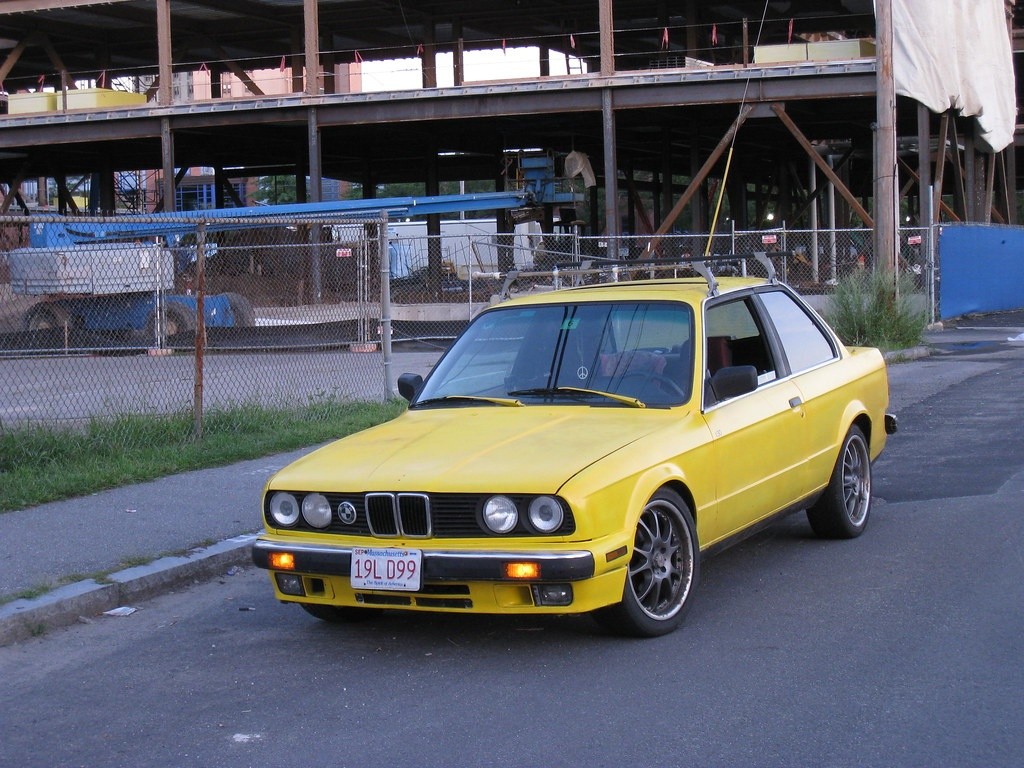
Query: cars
252,250,897,636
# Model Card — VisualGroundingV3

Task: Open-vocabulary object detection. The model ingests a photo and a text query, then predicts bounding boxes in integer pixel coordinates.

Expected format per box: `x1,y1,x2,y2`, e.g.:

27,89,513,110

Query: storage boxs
57,88,147,110
807,39,876,62
8,92,57,114
754,44,807,64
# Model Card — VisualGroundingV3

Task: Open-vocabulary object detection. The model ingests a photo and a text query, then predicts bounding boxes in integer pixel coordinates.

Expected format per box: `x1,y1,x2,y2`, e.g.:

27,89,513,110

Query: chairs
679,336,732,377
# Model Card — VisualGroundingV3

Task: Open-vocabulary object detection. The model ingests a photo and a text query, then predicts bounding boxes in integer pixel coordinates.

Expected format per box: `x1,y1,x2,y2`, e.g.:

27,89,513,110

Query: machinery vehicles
9,150,596,337
